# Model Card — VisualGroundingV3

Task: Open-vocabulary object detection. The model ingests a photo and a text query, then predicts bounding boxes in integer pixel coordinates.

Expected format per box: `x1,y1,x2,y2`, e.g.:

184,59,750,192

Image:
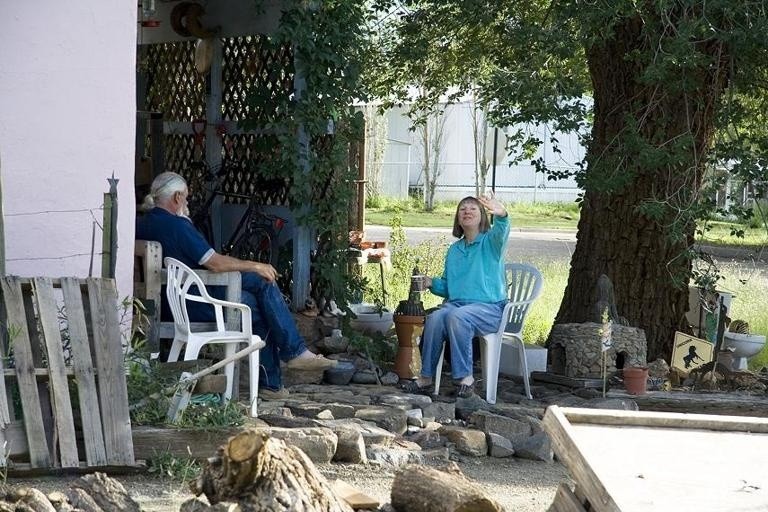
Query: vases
618,363,650,397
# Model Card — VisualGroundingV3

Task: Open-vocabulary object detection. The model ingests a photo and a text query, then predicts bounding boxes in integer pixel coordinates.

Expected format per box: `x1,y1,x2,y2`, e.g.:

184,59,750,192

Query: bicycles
188,157,288,275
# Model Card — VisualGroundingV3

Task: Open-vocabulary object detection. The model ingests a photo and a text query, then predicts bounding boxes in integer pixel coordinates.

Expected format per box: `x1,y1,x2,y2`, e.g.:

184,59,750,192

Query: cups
411,275,427,293
621,368,649,394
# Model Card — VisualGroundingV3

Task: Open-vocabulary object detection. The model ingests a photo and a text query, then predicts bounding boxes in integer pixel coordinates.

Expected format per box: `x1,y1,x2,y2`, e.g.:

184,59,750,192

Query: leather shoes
285,353,340,371
258,385,292,401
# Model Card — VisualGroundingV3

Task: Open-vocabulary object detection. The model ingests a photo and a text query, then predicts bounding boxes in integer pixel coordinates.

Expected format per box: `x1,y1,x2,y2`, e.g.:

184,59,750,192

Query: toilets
686,285,766,369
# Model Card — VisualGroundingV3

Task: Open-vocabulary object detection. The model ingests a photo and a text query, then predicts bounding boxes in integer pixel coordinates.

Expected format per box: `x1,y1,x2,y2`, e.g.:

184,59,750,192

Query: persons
134,172,338,401
401,190,511,398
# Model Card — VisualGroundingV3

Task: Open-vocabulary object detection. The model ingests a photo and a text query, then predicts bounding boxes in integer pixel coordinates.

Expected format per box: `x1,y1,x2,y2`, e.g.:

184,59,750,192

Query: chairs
134,237,248,404
154,253,266,422
432,261,544,405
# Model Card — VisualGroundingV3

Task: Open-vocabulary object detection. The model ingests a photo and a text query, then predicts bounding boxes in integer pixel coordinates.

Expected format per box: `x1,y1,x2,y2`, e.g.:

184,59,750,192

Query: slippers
457,377,477,399
400,380,434,394
